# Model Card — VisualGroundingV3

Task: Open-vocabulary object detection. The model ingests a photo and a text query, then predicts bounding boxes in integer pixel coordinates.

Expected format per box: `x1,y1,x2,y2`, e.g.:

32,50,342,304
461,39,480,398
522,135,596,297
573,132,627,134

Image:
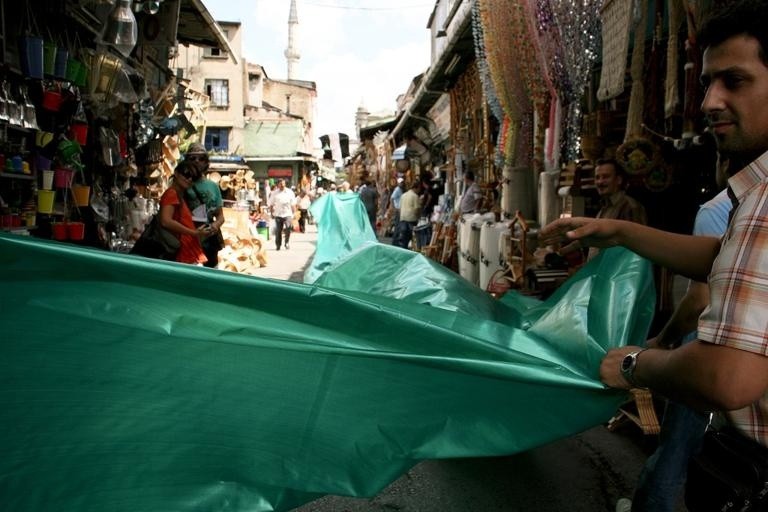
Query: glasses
190,155,205,161
180,171,198,183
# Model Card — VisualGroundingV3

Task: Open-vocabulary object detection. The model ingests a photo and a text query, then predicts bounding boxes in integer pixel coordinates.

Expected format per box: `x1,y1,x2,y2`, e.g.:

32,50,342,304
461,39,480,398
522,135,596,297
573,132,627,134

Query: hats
183,142,208,159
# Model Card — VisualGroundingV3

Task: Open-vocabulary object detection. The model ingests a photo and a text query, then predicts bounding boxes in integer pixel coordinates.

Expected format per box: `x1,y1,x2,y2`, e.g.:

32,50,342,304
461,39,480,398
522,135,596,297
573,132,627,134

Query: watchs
620,347,648,389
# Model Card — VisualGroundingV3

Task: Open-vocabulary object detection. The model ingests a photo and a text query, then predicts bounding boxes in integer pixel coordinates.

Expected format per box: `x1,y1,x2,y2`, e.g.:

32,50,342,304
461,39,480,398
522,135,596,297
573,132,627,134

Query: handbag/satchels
128,186,184,260
212,227,225,251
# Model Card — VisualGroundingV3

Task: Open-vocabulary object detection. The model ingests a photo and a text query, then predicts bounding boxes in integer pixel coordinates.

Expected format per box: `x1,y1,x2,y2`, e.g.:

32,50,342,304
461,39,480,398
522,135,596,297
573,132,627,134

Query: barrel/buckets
38,82,90,240
480,221,513,292
457,212,496,283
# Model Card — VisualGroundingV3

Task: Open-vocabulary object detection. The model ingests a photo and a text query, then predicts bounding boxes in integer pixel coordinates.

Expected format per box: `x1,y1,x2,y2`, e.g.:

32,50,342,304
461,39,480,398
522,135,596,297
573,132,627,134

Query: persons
266,168,438,252
158,159,218,264
614,146,734,512
536,13,768,512
585,158,649,265
182,142,225,268
